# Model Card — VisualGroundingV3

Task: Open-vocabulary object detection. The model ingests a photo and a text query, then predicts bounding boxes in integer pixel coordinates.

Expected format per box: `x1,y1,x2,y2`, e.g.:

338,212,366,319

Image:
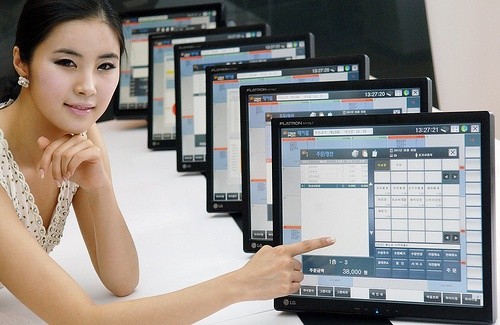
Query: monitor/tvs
111,2,226,120
148,23,272,147
272,110,497,325
238,77,433,254
206,53,369,212
173,32,314,173
228,103,260,169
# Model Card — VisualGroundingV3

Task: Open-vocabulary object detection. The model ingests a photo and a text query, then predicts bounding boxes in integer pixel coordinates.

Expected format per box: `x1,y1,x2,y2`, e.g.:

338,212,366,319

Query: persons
0,1,338,325
